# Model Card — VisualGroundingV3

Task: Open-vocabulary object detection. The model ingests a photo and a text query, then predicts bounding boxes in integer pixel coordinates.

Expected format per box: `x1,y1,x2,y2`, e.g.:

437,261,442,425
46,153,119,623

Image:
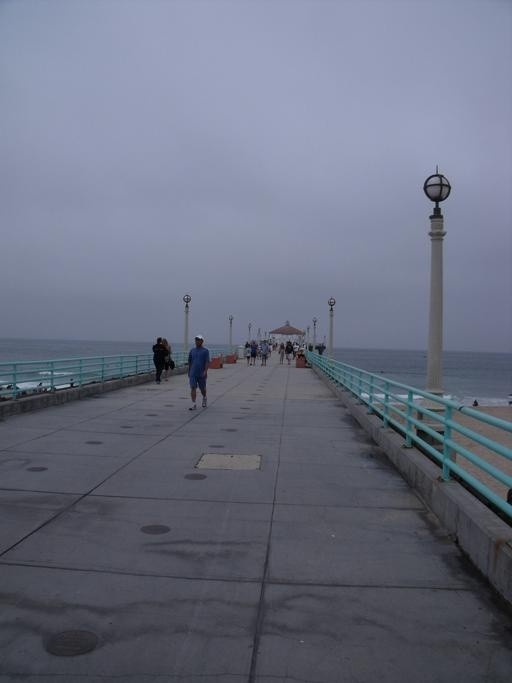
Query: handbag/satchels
169,359,176,370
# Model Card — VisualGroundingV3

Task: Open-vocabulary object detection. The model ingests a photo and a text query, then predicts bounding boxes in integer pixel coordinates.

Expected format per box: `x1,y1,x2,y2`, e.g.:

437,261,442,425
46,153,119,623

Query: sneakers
202,399,207,407
189,403,197,410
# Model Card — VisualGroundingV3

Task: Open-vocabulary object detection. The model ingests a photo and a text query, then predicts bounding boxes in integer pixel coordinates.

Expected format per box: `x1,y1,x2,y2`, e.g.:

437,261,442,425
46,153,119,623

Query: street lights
422,164,452,435
302,317,318,353
247,322,272,343
183,294,191,352
327,294,336,368
229,314,234,351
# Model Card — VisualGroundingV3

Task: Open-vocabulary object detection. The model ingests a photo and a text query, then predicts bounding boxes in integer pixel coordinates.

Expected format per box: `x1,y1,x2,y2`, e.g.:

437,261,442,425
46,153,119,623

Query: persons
152,336,169,384
188,334,210,409
244,337,298,367
162,337,175,381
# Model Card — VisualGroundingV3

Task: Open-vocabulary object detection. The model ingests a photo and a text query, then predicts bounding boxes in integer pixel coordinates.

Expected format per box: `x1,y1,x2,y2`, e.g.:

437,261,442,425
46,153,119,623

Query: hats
195,335,204,341
